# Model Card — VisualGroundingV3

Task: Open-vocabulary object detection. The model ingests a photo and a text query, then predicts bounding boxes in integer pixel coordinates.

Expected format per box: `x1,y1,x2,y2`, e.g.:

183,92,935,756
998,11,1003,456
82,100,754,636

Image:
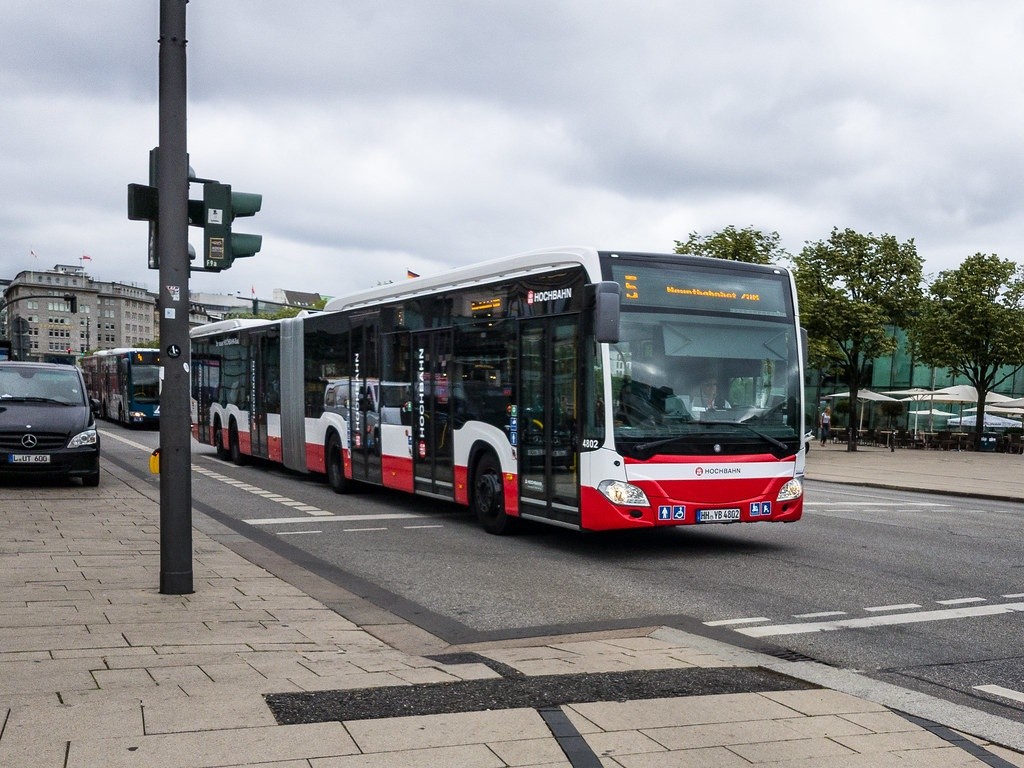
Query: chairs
830,428,1024,454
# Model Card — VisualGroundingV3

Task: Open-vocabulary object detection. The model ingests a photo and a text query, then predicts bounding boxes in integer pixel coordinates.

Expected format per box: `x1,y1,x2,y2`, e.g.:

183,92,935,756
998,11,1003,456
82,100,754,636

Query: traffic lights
204,182,263,270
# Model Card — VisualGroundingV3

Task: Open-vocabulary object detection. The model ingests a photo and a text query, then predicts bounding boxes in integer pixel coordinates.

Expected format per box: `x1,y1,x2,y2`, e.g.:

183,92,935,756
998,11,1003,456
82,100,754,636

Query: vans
0,361,101,486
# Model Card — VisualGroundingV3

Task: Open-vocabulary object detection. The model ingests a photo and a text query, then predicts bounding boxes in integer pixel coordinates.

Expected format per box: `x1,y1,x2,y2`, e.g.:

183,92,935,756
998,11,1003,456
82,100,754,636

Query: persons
818,406,832,447
628,376,662,426
689,376,732,421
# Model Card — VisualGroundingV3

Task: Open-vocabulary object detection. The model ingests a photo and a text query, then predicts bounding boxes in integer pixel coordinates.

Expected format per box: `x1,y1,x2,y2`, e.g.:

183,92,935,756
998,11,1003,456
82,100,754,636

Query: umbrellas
819,383,1024,454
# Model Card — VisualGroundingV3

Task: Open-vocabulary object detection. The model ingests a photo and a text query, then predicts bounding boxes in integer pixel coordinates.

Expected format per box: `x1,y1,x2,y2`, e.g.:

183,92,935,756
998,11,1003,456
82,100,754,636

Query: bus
79,347,161,427
190,246,810,536
